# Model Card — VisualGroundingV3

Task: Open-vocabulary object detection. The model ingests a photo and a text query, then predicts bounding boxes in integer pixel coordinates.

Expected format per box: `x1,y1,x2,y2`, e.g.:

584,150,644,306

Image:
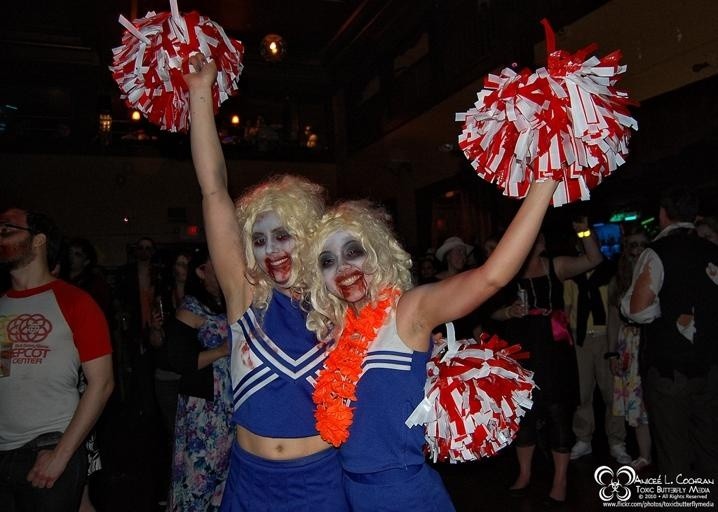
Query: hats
436,236,475,261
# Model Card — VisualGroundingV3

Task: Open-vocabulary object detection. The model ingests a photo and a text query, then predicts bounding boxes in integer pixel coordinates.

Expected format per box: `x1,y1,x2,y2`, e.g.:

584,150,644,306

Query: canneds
517,289,529,316
154,296,164,321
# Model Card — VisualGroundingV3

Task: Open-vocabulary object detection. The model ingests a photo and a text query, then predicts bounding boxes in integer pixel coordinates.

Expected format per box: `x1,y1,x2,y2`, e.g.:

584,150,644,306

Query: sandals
630,457,652,472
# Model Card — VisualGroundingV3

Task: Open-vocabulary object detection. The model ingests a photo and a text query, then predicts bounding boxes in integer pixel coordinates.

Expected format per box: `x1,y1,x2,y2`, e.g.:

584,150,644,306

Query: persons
179,51,350,512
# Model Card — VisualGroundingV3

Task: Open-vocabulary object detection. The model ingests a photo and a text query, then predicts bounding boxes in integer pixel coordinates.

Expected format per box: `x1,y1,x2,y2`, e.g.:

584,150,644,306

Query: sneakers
508,476,532,497
610,442,632,464
570,440,593,460
